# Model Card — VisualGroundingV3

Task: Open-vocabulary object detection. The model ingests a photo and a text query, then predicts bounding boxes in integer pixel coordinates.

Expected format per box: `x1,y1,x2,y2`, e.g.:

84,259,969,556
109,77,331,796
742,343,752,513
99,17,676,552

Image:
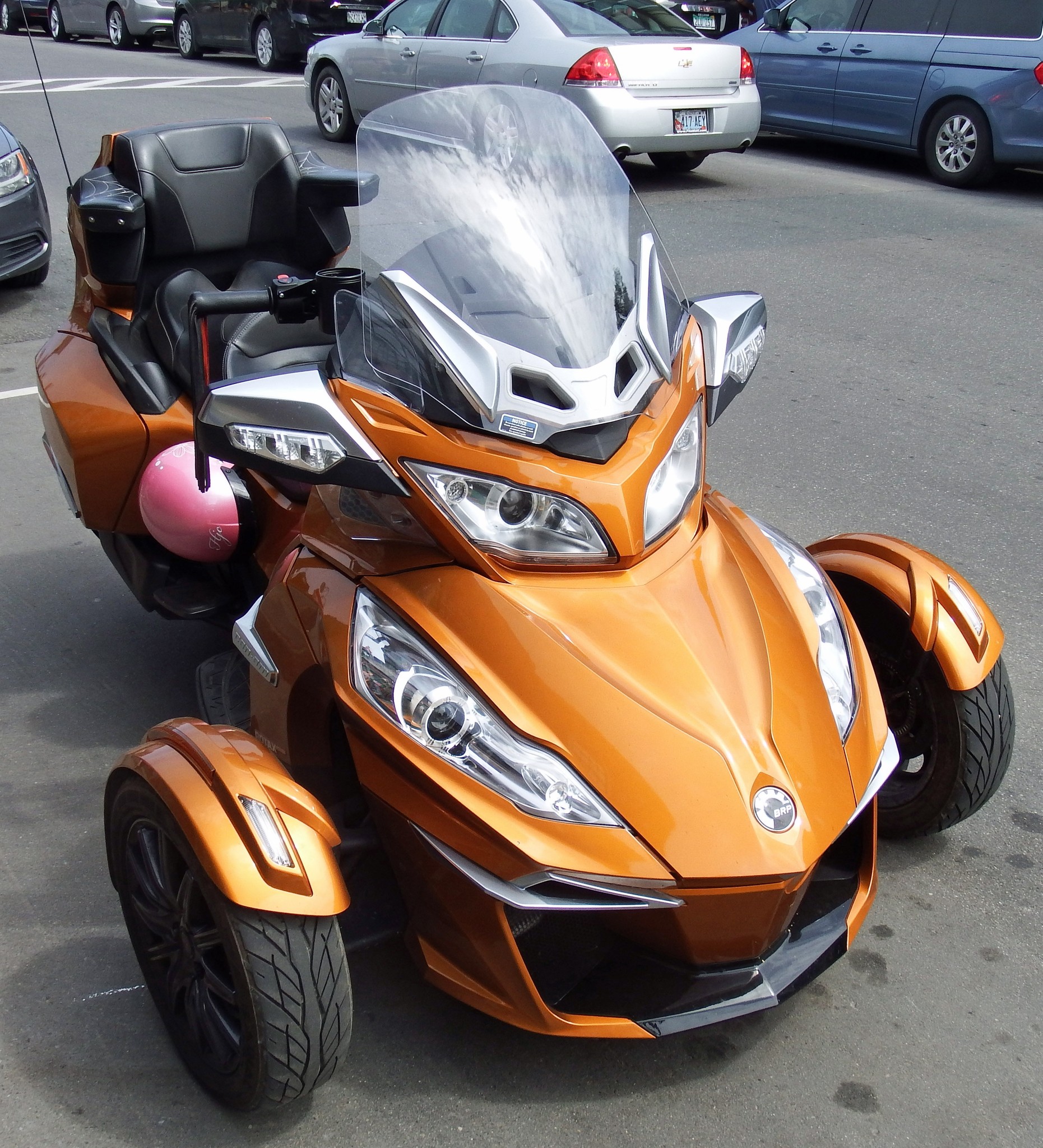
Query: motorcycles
28,82,1020,1122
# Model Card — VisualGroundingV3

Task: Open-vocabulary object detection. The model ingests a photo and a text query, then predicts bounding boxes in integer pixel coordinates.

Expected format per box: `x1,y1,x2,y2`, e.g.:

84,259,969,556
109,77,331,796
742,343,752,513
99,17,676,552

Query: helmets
138,441,255,563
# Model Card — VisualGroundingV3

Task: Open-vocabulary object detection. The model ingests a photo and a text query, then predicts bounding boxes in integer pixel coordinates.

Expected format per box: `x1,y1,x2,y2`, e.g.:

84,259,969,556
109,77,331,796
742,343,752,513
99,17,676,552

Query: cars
47,0,176,51
0,120,52,286
304,0,763,172
0,0,53,38
713,0,1043,189
171,0,396,72
610,0,757,40
